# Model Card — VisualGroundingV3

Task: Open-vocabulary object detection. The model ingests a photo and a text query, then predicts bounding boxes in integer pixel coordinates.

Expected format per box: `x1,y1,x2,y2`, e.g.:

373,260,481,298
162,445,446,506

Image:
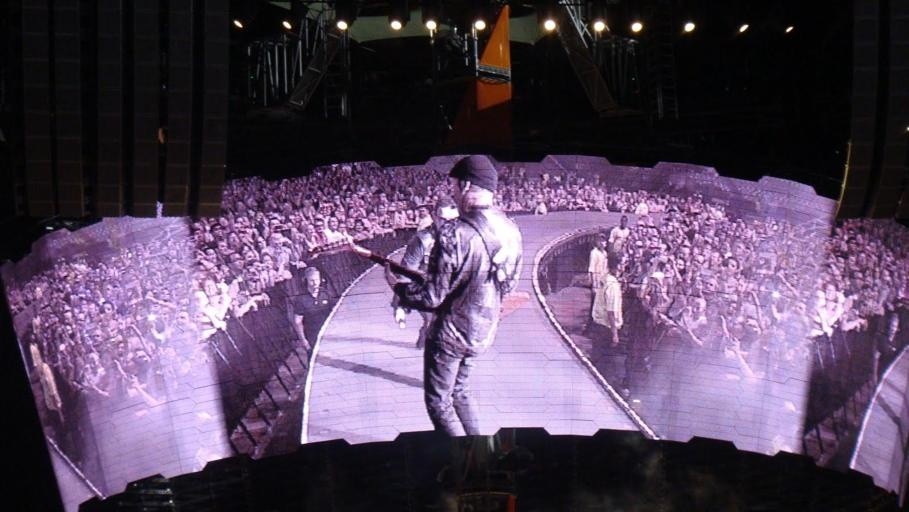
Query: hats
448,151,499,195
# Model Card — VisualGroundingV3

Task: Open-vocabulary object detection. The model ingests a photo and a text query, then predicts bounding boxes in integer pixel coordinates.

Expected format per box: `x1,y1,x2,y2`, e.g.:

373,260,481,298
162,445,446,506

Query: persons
6,161,460,447
493,165,908,453
384,153,522,439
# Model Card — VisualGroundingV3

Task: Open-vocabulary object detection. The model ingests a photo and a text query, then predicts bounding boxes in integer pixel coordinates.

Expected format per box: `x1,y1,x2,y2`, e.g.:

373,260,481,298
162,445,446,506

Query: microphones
398,318,406,329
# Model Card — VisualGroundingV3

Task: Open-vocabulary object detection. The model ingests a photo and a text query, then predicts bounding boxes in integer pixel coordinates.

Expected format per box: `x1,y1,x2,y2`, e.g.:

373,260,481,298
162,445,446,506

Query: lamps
226,0,798,40
